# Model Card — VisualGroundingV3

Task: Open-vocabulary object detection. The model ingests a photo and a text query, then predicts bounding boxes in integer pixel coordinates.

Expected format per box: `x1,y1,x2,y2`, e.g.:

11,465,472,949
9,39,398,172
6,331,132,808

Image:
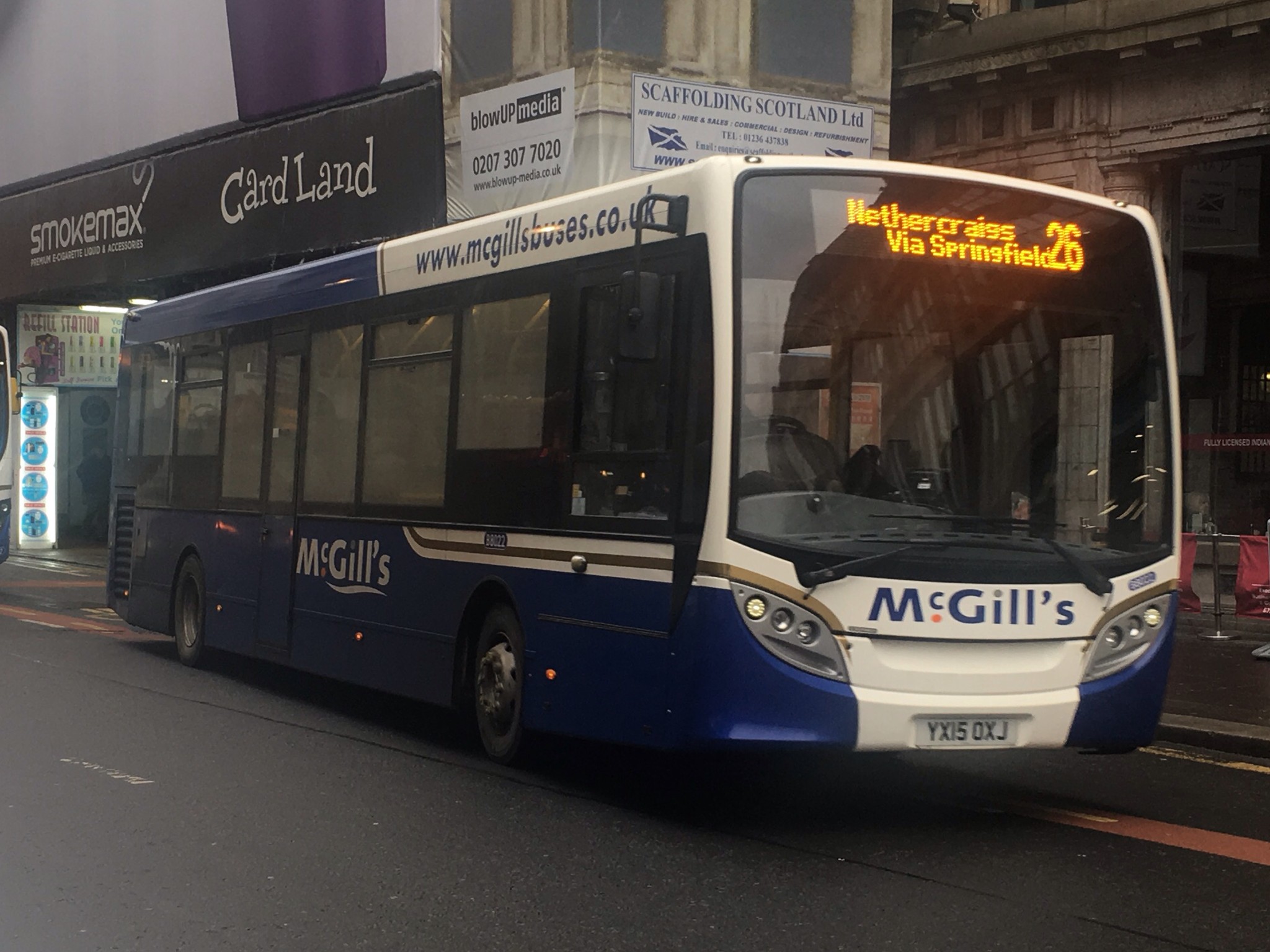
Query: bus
108,156,1181,783
0,326,24,564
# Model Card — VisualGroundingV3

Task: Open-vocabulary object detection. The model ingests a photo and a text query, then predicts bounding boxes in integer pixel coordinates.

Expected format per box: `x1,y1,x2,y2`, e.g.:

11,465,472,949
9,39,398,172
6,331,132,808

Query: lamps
127,299,159,305
947,0,984,23
77,305,130,314
